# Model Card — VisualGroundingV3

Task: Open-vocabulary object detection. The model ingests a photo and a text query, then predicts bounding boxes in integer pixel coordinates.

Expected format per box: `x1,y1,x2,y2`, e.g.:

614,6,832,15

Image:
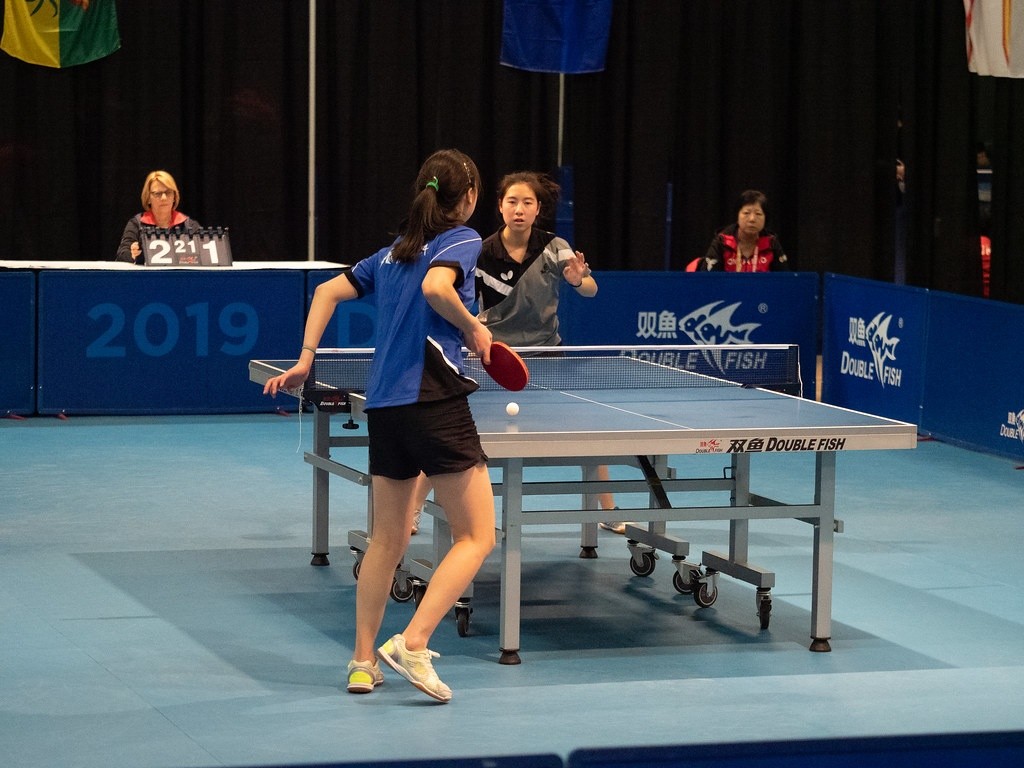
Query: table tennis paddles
481,341,530,391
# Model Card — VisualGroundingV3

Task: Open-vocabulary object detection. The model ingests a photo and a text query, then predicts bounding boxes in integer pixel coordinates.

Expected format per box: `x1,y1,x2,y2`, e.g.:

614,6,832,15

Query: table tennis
505,402,519,415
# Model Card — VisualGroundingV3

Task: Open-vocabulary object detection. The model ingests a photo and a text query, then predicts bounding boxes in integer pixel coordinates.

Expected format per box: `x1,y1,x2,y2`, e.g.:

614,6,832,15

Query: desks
0,260,354,417
248,356,916,665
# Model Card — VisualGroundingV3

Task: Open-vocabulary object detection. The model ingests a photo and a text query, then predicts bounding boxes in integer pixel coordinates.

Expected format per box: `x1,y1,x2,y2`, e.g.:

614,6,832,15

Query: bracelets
564,279,583,287
303,346,314,352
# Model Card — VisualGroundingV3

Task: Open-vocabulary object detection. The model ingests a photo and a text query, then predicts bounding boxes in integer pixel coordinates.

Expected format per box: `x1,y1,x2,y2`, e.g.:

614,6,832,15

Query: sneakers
412,508,420,533
347,658,384,691
378,634,452,701
599,506,635,534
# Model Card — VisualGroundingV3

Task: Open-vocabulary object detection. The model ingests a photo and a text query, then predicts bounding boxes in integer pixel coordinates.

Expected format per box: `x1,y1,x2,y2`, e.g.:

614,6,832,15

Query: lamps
220,76,255,108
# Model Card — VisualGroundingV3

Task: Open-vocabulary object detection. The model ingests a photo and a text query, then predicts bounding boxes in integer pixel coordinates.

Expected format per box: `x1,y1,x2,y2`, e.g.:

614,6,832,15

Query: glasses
150,189,172,198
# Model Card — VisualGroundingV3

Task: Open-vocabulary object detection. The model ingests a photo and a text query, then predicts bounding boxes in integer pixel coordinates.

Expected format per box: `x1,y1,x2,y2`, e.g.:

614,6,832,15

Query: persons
261,148,491,700
474,171,596,355
696,188,793,274
116,170,203,264
